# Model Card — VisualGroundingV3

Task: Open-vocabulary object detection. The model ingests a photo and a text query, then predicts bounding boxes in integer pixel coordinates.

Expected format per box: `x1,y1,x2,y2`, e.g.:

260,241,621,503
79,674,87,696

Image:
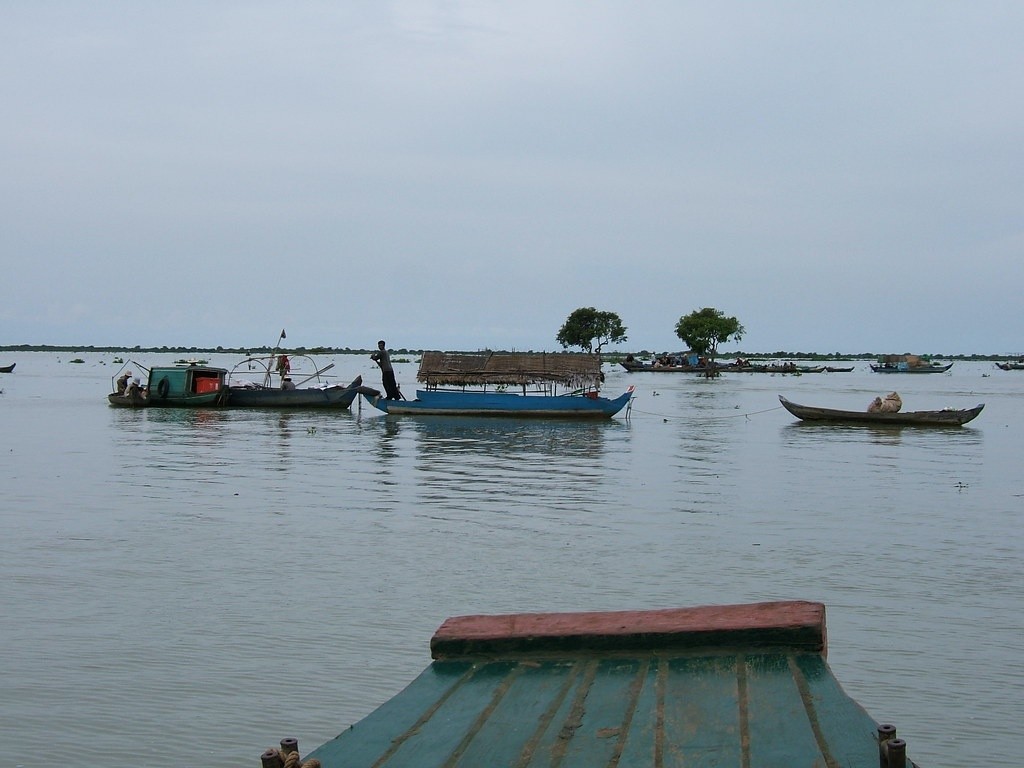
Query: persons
880,391,903,413
117,370,132,395
868,397,883,413
735,357,751,367
124,377,140,396
654,356,670,368
276,356,291,388
651,351,657,365
371,341,401,401
283,378,295,390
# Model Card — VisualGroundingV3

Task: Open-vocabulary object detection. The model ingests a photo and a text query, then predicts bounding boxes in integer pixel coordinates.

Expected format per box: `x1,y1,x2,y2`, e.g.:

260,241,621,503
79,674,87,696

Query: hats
133,377,140,384
126,370,132,376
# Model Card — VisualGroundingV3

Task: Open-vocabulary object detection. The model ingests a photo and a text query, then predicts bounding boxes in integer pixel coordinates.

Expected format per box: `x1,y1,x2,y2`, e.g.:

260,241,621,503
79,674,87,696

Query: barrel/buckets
196,377,222,393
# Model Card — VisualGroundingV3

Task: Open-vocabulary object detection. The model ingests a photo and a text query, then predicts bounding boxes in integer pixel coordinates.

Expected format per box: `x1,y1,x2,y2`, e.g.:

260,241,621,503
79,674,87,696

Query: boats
621,357,826,374
779,395,984,427
0,363,16,373
870,360,953,373
109,355,362,409
357,384,634,418
995,363,1024,370
825,367,854,372
